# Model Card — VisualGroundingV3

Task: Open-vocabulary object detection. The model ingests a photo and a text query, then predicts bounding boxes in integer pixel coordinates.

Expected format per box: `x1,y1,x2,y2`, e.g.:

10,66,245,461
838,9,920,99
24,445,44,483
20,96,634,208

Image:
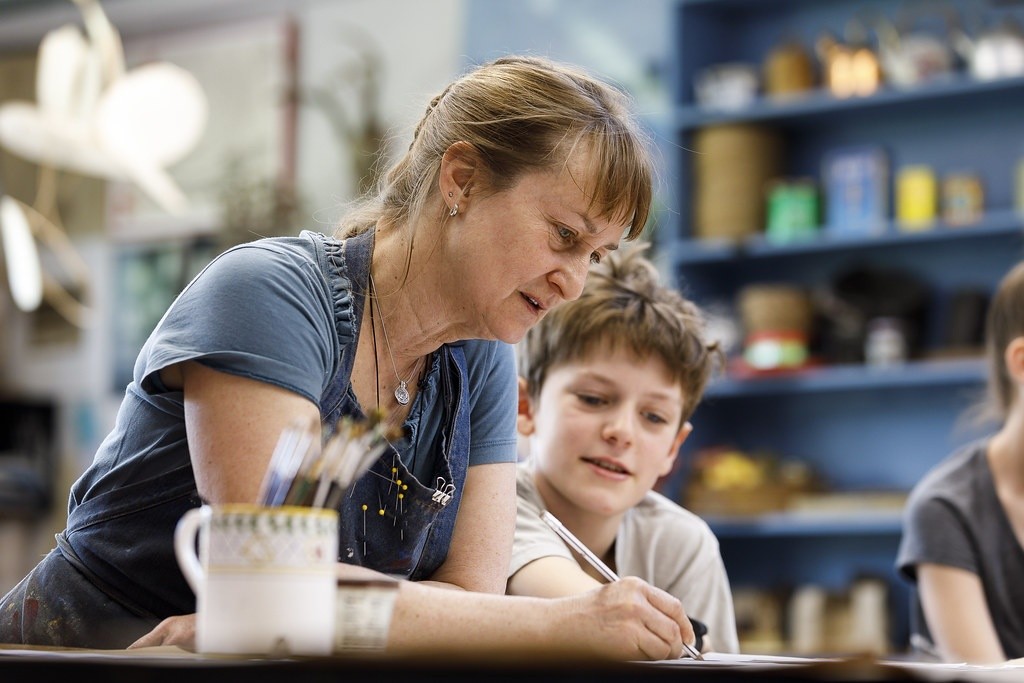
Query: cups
338,579,399,652
174,504,339,653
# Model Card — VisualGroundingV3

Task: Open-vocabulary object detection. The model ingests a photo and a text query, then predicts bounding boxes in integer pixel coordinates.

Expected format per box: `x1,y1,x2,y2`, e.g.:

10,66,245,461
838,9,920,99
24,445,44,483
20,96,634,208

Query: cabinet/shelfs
667,1,1024,644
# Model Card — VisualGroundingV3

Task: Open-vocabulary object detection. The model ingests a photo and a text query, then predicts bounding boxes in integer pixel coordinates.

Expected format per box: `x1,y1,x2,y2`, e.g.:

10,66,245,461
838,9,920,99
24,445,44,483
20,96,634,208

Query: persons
505,241,740,654
894,260,1024,666
1,55,695,662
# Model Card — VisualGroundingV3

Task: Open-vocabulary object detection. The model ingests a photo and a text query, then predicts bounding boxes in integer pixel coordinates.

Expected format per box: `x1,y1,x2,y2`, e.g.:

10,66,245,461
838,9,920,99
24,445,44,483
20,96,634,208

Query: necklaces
369,276,429,474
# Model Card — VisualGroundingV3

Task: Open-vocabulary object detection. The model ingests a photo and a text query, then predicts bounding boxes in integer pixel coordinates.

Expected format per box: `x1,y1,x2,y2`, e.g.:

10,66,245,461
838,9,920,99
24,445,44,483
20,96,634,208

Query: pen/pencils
539,509,705,660
250,418,388,510
